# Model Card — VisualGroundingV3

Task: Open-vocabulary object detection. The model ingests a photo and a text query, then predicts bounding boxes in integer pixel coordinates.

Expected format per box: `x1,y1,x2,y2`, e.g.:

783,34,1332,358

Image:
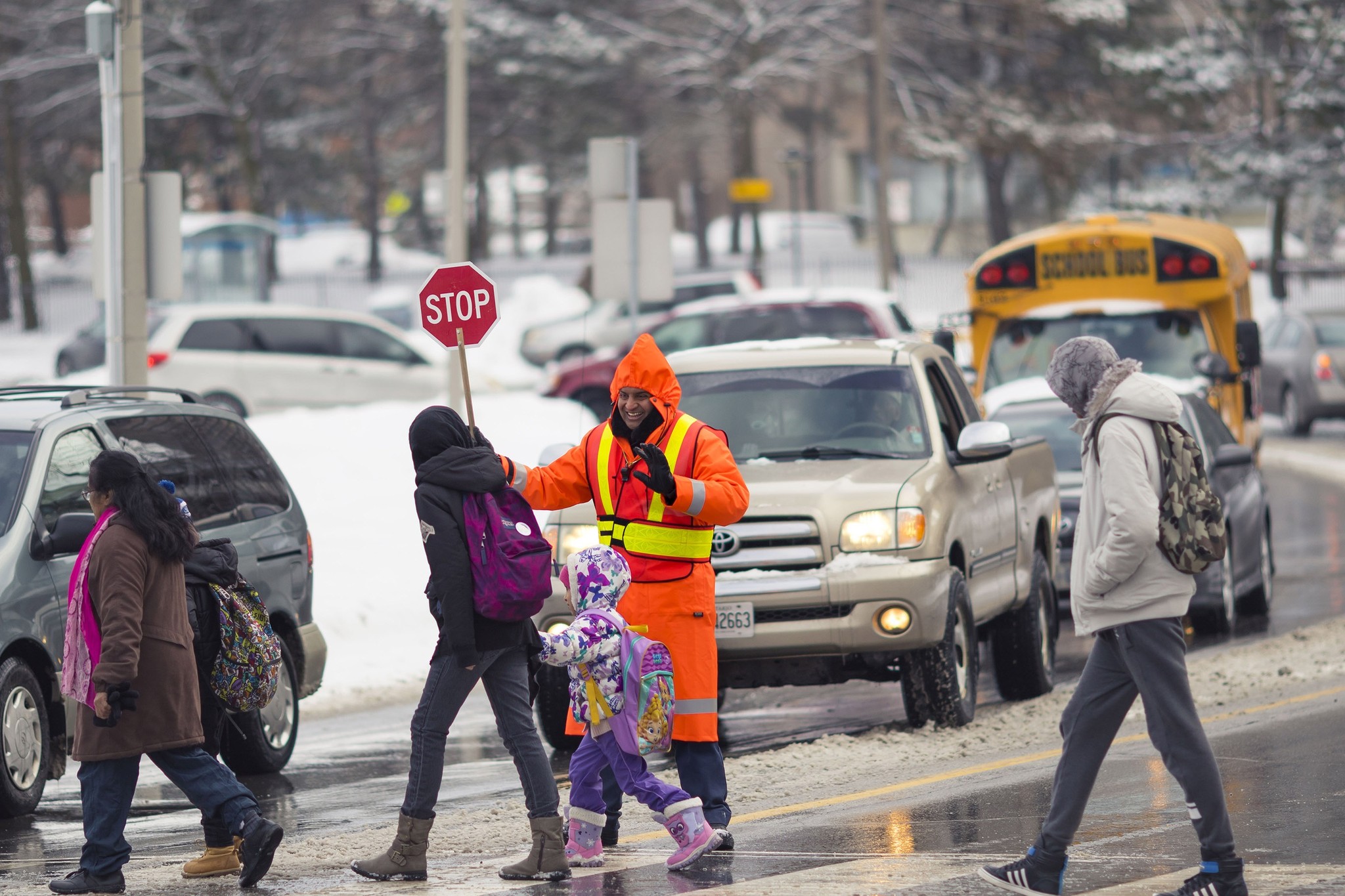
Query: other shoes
49,868,125,894
562,820,619,845
239,818,283,887
709,823,734,849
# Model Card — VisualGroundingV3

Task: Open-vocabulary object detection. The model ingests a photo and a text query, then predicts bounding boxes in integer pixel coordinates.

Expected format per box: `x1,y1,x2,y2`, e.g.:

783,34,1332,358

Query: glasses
81,488,100,501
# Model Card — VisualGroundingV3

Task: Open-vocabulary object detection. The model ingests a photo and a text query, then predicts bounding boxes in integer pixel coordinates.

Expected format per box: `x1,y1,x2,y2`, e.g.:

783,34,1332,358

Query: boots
498,816,572,881
351,808,436,880
650,796,723,871
563,804,607,867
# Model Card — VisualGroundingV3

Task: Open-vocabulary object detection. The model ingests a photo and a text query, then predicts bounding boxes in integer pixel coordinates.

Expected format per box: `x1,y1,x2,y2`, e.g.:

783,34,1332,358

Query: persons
350,405,571,882
157,480,281,878
48,450,283,895
538,544,723,871
977,336,1248,896
496,333,749,849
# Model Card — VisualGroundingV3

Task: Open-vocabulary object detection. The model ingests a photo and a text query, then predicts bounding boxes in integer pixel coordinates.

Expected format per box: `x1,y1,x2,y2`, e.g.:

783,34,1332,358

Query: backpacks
462,483,552,622
576,608,676,756
198,577,282,712
1094,412,1226,575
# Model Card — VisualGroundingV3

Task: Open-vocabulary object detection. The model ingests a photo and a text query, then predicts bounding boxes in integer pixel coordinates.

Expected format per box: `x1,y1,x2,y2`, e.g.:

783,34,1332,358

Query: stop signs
418,262,500,349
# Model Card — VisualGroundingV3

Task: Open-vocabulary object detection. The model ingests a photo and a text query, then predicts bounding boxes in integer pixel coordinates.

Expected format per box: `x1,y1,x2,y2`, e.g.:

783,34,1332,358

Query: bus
929,208,1263,457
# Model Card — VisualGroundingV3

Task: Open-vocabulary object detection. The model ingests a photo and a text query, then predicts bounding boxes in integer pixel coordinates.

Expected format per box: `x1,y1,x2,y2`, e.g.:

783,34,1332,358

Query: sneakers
181,839,242,878
978,845,1068,896
1153,858,1248,896
232,836,245,863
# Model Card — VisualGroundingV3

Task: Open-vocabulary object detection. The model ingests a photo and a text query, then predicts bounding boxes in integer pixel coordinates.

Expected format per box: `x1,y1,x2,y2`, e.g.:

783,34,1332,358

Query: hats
158,480,192,524
559,565,570,589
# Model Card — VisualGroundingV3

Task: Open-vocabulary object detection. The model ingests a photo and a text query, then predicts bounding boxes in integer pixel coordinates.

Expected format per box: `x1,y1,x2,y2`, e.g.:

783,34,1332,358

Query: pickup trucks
532,334,1066,753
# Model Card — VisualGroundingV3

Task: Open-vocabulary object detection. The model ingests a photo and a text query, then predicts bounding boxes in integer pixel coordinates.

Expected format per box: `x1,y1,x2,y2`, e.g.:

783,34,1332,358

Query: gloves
633,443,677,505
466,425,501,462
93,682,139,727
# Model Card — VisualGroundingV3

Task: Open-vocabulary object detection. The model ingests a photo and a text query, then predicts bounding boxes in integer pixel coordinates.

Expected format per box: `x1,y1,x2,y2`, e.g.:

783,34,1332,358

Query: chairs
833,391,903,439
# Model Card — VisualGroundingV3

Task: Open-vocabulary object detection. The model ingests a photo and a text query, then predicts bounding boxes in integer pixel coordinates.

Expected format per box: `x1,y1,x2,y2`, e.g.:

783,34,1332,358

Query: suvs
544,290,920,433
46,301,528,424
0,384,329,826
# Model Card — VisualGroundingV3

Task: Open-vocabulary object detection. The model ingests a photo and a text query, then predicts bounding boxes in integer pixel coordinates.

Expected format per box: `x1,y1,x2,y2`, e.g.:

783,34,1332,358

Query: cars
967,369,1276,643
522,272,765,369
1246,290,1345,436
702,209,890,302
52,303,168,379
